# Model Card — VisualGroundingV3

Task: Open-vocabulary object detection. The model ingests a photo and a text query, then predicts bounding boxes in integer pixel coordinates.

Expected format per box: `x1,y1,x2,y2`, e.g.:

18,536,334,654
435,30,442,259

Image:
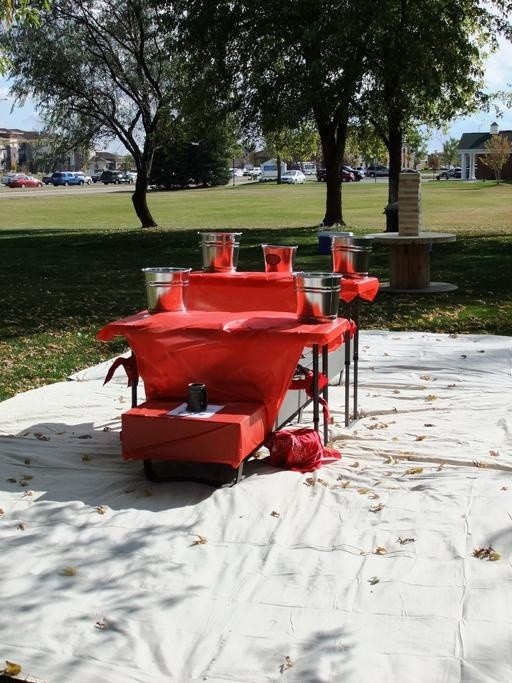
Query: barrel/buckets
292,271,343,324
328,233,375,279
142,267,192,313
198,232,243,272
261,244,298,272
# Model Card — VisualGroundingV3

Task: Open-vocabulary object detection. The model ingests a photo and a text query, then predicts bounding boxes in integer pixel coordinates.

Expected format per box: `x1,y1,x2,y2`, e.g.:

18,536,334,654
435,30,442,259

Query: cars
280,171,306,184
316,166,390,182
0,168,137,188
402,168,421,174
231,168,261,177
439,163,477,178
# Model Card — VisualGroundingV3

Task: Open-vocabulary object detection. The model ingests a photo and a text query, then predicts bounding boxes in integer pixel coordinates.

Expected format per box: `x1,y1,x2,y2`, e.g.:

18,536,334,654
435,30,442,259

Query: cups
187,382,209,413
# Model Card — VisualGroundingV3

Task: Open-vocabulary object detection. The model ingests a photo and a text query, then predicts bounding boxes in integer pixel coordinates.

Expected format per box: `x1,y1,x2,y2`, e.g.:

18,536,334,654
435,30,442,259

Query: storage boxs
317,231,354,255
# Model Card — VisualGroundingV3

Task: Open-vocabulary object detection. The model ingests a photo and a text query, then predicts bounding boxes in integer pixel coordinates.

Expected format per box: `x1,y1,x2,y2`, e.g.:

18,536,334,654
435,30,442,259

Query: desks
110,309,350,446
183,270,379,428
121,399,266,488
364,232,459,294
